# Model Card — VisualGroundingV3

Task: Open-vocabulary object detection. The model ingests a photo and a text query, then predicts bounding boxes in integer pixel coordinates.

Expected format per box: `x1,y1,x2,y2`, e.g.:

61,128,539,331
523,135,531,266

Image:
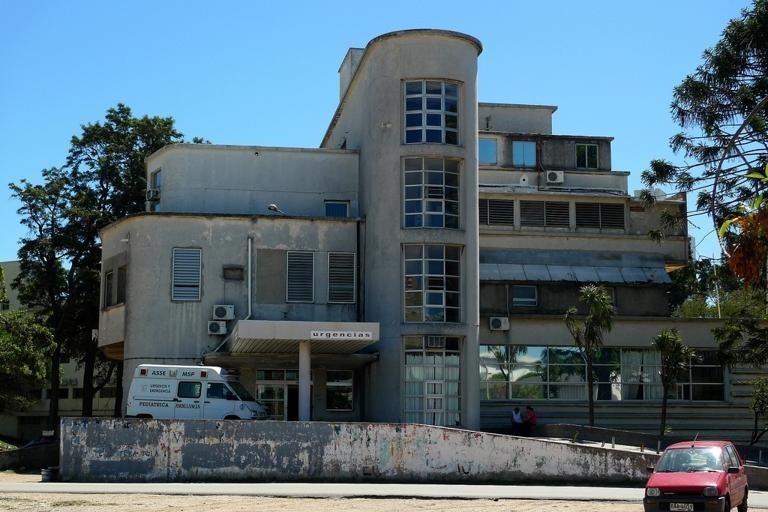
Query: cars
644,441,748,512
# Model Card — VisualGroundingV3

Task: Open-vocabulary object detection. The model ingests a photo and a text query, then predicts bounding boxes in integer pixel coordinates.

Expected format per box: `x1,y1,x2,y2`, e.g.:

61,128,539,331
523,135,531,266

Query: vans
126,364,267,422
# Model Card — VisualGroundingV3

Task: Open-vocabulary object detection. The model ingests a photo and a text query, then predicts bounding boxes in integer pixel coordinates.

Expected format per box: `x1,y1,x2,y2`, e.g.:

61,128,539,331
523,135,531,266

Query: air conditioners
489,317,510,331
212,305,235,320
208,321,227,334
546,170,564,184
145,188,160,201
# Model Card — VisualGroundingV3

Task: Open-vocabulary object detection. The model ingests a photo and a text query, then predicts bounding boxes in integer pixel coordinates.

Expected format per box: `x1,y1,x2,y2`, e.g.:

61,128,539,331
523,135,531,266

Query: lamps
267,203,287,216
